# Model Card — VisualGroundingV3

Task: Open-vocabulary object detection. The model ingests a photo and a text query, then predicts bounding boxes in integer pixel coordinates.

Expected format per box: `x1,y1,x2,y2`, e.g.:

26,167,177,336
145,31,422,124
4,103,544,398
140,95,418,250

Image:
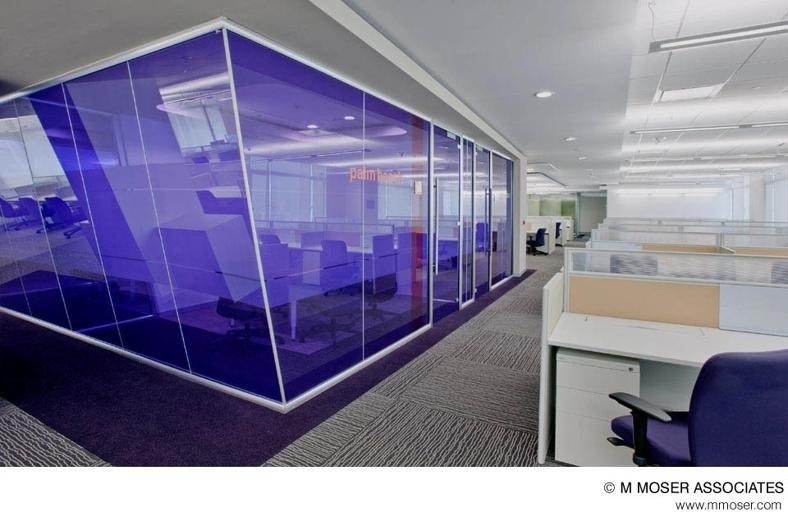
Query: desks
255,215,506,340
537,312,788,465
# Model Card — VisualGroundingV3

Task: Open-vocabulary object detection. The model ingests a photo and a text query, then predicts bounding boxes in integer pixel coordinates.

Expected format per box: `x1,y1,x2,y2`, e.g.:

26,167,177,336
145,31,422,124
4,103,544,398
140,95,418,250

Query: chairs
555,222,563,247
526,228,548,257
0,197,82,239
607,348,788,467
